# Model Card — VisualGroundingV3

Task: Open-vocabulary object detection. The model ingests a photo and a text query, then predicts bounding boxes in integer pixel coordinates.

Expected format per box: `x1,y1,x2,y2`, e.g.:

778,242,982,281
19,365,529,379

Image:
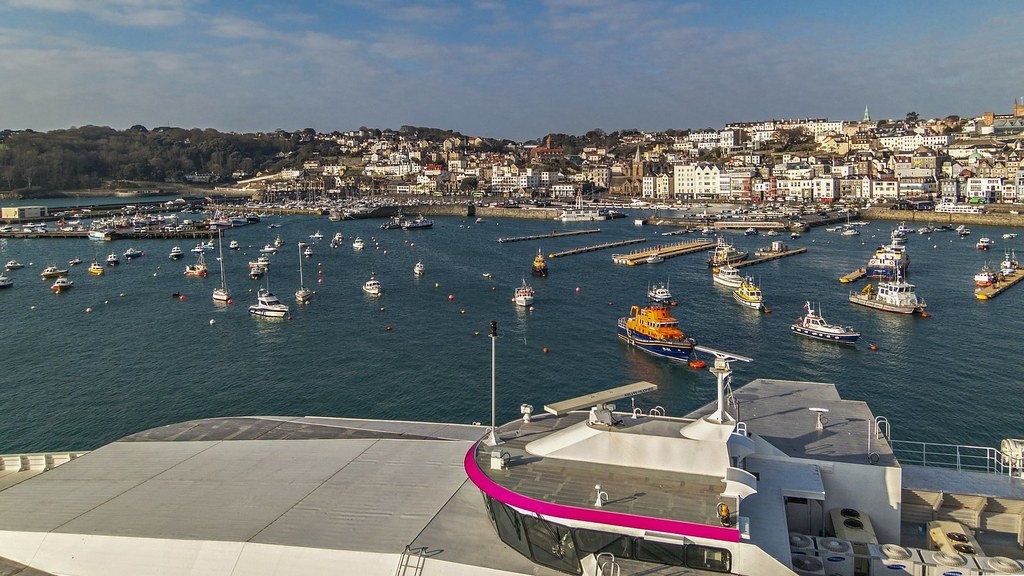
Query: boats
707,232,750,269
790,300,862,346
848,258,928,315
732,271,764,312
976,236,990,250
973,247,1020,287
462,320,1023,575
617,274,697,365
889,220,972,245
712,255,745,289
553,195,607,223
0,194,553,324
864,235,911,281
